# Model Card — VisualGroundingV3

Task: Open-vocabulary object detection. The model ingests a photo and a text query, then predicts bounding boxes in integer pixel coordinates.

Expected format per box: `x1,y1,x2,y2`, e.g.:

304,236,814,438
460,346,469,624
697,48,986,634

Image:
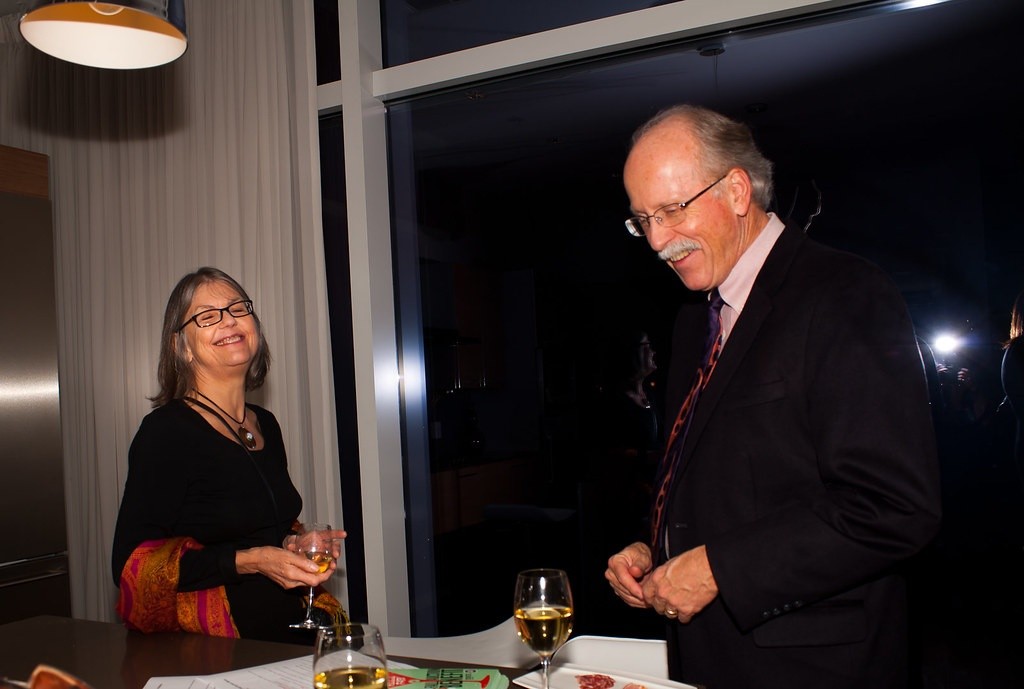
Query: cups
312,623,386,689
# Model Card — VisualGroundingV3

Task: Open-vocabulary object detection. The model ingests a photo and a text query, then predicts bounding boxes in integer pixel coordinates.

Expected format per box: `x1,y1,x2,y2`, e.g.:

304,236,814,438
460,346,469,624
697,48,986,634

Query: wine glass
515,569,574,689
288,521,334,629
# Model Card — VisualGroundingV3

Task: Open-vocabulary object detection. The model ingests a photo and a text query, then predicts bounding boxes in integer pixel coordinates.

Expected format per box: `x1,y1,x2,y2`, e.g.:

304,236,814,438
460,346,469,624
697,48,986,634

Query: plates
512,666,698,689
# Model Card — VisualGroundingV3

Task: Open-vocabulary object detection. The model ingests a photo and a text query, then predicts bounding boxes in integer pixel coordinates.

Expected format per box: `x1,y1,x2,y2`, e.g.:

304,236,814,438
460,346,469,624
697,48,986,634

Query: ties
651,288,724,567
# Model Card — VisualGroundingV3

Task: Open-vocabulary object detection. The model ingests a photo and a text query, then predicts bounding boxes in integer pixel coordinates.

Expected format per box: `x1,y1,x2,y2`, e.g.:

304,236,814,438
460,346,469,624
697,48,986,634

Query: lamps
20,0,189,69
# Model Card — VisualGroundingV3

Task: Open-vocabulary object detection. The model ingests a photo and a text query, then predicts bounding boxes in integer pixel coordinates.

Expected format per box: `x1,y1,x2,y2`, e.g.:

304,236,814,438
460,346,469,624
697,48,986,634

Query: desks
0,613,704,689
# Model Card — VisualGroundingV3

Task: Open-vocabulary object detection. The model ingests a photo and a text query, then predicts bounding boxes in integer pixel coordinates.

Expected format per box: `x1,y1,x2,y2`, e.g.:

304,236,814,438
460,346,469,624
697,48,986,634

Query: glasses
175,300,253,331
625,174,727,237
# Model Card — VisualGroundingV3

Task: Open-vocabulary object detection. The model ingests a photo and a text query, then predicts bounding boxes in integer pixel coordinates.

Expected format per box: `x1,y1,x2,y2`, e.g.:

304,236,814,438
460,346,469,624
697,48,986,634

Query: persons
605,106,939,688
921,301,1023,415
111,265,346,645
626,334,659,403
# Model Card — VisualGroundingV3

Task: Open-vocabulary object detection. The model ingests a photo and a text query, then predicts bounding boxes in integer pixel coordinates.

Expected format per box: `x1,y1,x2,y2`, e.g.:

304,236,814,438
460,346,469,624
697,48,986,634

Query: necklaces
192,388,257,450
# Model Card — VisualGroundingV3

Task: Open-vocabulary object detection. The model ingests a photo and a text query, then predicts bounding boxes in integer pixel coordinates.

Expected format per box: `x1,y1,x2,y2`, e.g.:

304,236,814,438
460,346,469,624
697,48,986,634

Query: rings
666,608,677,616
